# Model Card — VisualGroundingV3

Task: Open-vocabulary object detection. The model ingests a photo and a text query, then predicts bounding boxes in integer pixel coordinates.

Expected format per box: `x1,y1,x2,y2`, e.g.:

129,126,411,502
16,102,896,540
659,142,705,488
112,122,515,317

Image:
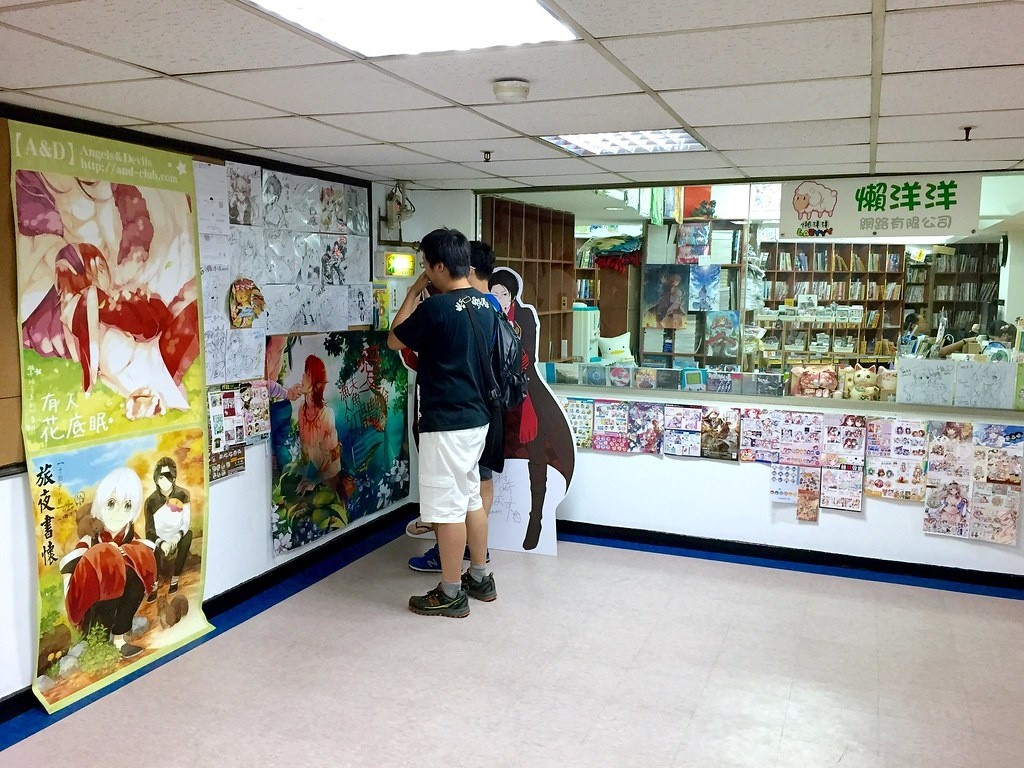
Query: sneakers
409,582,470,618
461,567,497,602
408,543,442,572
463,544,490,563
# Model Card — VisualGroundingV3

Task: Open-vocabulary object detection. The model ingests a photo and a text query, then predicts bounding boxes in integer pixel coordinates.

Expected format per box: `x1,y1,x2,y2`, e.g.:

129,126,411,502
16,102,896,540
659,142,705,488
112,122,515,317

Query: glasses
420,261,426,268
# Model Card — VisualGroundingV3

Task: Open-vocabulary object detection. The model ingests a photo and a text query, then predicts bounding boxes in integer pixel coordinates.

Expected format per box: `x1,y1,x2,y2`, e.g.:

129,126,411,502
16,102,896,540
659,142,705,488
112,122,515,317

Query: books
576,246,1024,414
537,361,556,384
556,363,578,384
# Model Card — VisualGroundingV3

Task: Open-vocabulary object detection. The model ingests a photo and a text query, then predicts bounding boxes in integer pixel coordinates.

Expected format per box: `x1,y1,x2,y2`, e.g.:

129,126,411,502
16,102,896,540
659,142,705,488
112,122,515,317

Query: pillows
596,331,633,359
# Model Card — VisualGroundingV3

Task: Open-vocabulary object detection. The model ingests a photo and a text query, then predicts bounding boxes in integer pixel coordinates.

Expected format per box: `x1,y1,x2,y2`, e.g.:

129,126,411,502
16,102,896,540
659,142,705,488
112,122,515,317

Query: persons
406,240,504,574
386,225,506,618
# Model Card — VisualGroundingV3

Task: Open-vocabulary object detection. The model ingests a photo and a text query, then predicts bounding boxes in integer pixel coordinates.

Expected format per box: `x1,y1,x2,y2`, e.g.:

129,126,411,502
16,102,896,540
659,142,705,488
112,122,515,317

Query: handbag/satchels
479,395,505,473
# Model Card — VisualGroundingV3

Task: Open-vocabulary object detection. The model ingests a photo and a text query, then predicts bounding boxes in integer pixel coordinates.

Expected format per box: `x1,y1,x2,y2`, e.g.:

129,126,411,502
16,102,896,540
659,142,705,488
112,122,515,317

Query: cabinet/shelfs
599,259,640,365
760,239,907,368
481,196,578,365
573,236,600,307
911,244,1000,341
639,216,761,378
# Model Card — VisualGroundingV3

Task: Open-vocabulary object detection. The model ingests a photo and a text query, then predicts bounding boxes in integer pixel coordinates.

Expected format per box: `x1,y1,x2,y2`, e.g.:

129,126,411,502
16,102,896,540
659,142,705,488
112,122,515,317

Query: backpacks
490,303,528,406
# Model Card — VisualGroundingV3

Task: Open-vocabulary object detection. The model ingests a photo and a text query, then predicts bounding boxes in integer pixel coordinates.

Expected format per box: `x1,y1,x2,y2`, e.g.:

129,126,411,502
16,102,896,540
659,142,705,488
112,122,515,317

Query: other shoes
147,584,178,603
120,644,143,657
